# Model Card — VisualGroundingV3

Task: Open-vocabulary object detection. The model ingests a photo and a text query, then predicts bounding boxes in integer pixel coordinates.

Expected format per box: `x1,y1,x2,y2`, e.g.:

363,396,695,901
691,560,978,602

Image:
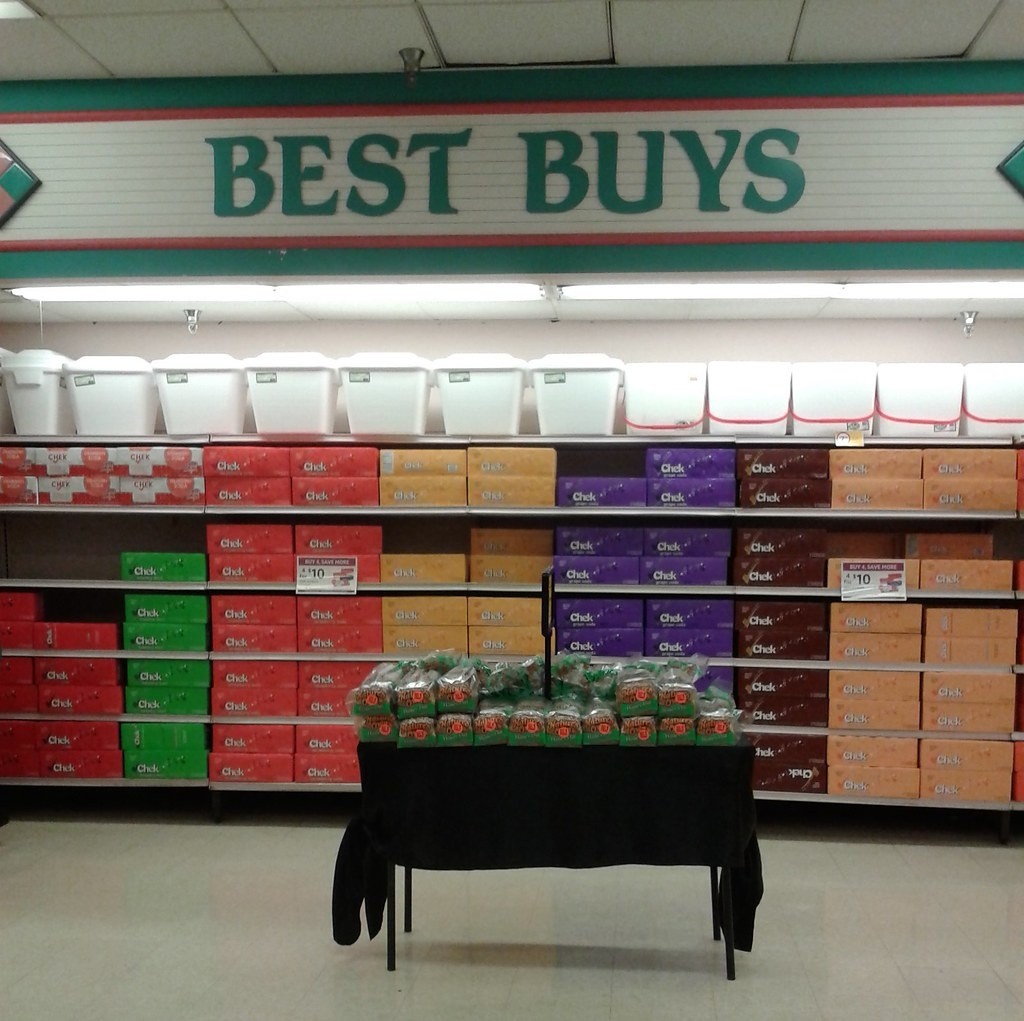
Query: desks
357,731,755,981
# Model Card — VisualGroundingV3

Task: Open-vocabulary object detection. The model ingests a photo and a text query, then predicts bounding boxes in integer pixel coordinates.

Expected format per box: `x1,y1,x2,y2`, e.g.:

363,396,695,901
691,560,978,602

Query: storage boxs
708,360,792,436
791,361,877,437
63,356,159,436
0,349,76,436
152,354,247,434
529,353,624,435
872,363,964,437
337,352,435,435
624,362,707,435
959,363,1024,436
245,352,342,435
433,353,529,436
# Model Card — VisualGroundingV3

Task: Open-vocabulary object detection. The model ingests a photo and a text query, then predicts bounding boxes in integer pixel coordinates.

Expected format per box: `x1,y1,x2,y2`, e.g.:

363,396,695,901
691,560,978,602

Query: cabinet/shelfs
0,437,1024,813
